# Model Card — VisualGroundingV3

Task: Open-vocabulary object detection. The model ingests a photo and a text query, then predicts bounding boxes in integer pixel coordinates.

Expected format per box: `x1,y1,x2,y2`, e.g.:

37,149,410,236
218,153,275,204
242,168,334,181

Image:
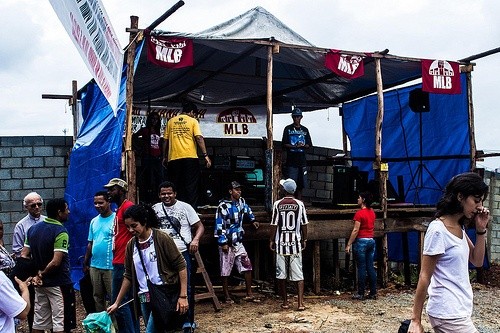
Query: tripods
402,111,444,203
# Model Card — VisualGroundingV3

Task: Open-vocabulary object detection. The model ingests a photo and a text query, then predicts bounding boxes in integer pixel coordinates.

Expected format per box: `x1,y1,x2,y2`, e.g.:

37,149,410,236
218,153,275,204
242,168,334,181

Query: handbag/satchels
146,279,189,333
185,243,199,274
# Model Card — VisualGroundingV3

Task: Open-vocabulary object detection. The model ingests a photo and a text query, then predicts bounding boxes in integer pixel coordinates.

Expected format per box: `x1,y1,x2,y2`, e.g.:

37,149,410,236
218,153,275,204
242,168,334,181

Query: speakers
409,88,430,112
332,165,358,206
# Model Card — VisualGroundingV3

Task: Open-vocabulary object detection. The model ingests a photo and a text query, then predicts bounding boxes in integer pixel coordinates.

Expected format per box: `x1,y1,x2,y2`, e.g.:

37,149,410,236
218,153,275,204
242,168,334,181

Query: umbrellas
82,297,135,333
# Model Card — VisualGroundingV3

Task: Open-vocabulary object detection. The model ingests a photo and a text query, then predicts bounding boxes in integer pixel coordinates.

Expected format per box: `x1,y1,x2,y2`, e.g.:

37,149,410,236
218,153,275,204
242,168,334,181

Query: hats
228,181,243,189
280,178,297,194
292,108,302,115
103,177,128,192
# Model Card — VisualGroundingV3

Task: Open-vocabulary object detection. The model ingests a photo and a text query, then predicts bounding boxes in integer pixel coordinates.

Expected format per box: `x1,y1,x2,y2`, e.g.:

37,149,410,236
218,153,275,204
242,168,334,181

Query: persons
83,190,118,333
406,172,491,333
0,219,33,333
20,197,70,333
151,181,205,333
106,201,189,333
344,192,377,301
282,109,314,201
12,192,49,262
162,105,212,211
213,181,260,304
268,178,310,311
103,178,141,333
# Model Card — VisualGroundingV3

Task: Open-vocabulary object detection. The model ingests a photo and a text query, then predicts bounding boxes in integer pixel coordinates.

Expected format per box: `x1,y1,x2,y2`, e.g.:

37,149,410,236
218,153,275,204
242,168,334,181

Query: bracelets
475,229,487,236
201,152,208,159
304,239,307,242
178,295,188,299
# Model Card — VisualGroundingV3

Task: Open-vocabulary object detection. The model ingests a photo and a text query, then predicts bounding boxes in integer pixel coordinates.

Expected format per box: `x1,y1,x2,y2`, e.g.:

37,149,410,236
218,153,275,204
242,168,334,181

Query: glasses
27,203,42,208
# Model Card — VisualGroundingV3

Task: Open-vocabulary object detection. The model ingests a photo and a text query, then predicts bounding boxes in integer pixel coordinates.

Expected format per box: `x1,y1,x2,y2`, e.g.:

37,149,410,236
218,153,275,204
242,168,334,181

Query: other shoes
226,298,235,304
281,304,289,309
246,296,260,303
352,294,365,300
366,294,376,300
298,304,305,310
